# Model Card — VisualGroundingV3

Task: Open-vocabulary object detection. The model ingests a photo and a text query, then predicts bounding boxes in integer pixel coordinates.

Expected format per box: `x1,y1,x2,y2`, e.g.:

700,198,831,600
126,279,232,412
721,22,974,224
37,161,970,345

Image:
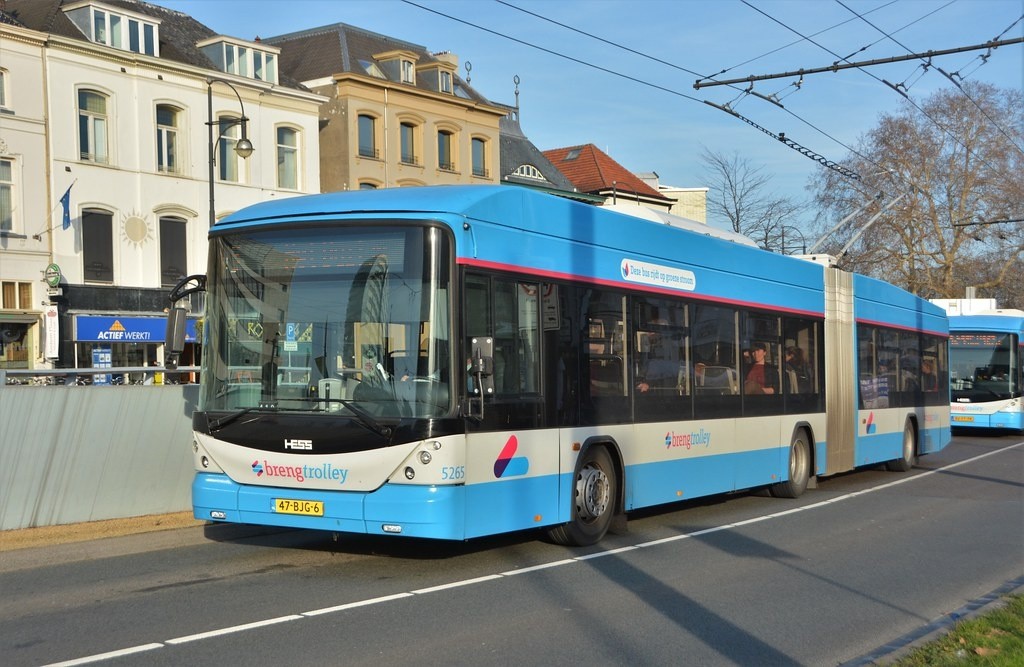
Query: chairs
677,365,798,395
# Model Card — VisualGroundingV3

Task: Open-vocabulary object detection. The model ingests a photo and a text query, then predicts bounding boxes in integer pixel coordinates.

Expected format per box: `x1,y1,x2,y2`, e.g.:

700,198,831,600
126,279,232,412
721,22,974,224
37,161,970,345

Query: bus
164,182,951,548
926,299,1023,433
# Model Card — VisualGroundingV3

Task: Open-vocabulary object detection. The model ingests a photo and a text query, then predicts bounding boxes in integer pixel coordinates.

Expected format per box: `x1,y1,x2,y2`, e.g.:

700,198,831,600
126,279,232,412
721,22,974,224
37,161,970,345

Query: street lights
204,76,260,233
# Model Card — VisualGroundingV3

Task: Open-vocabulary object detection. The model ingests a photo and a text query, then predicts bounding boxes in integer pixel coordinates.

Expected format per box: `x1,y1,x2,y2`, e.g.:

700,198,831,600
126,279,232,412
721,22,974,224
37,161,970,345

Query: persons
637,315,812,396
401,348,477,395
991,367,1009,380
